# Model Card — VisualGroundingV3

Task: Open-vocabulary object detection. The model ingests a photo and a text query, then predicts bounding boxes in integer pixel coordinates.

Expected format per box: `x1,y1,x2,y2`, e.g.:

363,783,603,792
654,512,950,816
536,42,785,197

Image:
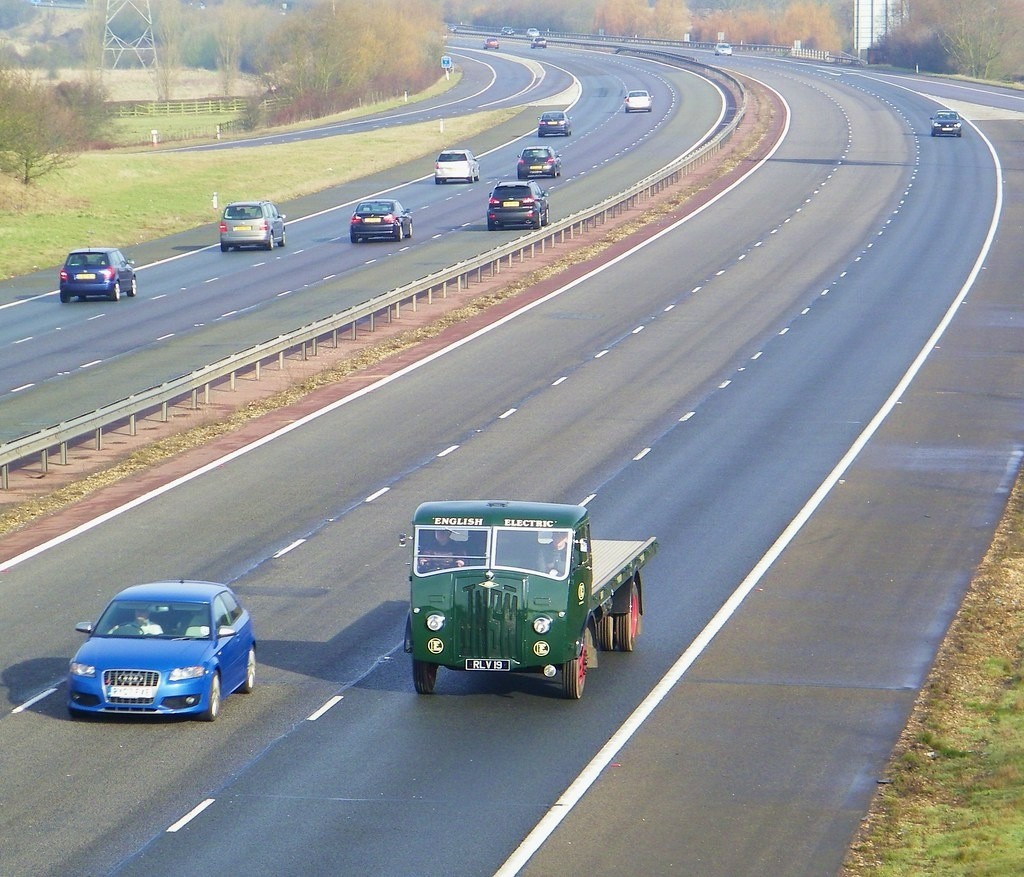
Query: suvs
220,199,287,251
517,145,563,179
487,179,549,231
434,149,479,185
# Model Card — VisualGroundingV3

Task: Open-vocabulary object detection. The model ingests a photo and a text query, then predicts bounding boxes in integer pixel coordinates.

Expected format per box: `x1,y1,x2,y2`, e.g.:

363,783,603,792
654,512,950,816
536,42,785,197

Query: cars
930,109,965,137
526,28,540,37
483,37,499,50
59,247,137,301
714,42,733,56
501,27,515,36
66,579,257,722
350,199,413,242
624,90,654,112
537,111,572,137
531,37,547,49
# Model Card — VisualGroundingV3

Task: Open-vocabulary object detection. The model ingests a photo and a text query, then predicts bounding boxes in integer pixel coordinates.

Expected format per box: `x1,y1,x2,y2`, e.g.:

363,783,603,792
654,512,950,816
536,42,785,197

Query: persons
419,531,468,567
106,609,164,635
535,531,568,576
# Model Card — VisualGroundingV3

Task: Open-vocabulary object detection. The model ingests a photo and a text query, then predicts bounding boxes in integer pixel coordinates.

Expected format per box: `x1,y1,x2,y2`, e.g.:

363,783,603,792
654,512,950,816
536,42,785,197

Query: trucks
403,499,659,700
